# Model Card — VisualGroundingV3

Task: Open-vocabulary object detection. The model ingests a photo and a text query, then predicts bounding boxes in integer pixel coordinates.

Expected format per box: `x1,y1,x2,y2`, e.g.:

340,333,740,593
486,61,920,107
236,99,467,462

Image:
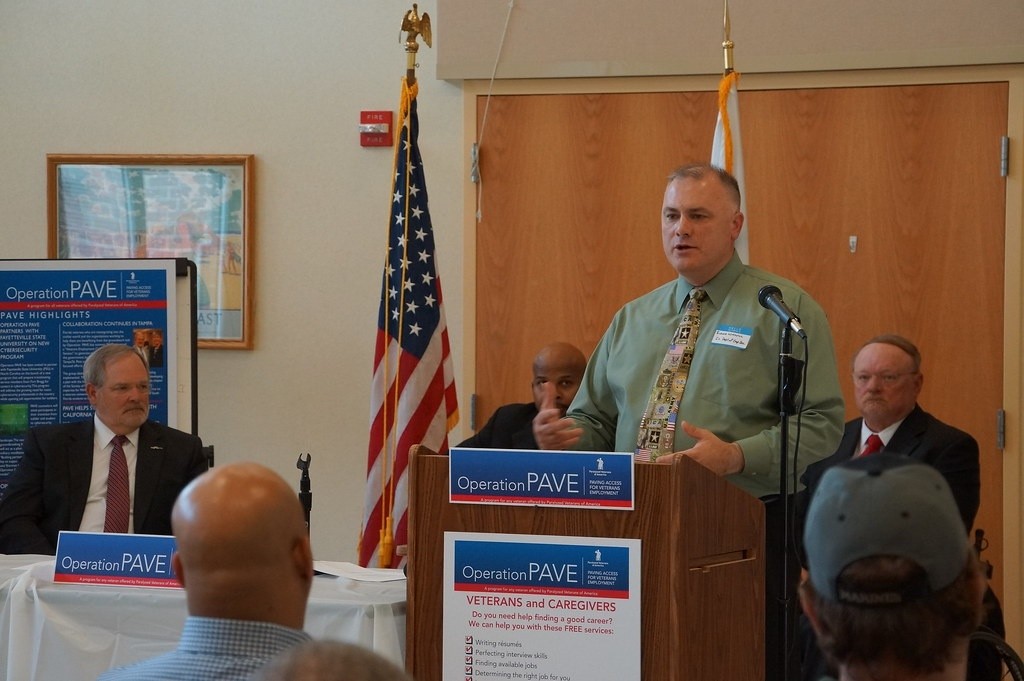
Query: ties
859,434,884,458
104,435,130,533
633,288,708,463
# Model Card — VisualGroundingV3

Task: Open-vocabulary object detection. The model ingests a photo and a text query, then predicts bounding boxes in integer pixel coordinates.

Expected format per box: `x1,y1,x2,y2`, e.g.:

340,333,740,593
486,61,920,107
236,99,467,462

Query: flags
359,79,459,569
710,73,749,265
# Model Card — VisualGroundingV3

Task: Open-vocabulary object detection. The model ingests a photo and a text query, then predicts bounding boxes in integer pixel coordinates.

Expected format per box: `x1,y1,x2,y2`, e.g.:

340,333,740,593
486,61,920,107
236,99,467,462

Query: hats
804,451,969,607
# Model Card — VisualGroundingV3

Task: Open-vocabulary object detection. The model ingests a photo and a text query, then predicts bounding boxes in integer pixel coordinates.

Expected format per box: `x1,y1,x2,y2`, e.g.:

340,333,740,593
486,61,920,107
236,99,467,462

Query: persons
0,344,208,556
794,455,983,681
800,335,980,681
443,343,587,455
535,164,846,681
134,331,163,367
97,461,409,681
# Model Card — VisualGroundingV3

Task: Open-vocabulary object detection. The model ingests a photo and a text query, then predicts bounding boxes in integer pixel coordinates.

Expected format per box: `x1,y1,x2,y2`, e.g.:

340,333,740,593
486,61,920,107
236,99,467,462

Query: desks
0,552,407,681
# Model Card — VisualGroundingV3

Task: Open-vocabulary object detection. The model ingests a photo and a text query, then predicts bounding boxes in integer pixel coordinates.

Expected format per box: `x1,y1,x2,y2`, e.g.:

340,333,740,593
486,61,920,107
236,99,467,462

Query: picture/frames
46,153,257,351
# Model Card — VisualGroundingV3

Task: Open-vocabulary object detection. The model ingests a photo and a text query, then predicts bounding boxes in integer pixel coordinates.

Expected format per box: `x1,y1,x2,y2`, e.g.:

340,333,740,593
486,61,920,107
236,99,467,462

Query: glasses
850,370,920,385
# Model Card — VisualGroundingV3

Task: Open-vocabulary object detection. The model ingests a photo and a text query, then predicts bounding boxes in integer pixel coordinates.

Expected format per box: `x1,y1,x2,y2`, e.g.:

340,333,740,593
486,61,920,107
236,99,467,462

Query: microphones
758,285,807,339
